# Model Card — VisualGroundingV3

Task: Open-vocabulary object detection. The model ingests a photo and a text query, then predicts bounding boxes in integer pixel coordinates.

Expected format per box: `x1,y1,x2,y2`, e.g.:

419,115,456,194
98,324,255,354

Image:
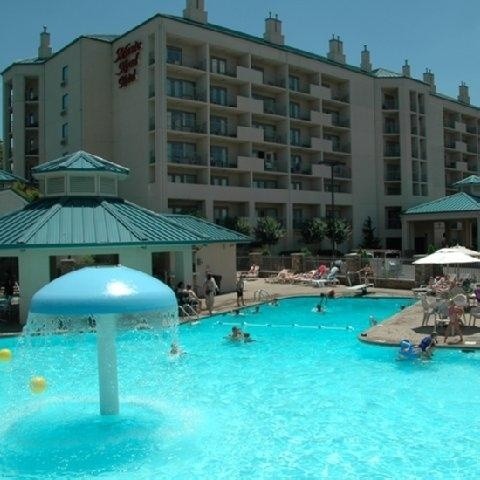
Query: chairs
264,270,340,288
412,277,480,334
174,288,202,316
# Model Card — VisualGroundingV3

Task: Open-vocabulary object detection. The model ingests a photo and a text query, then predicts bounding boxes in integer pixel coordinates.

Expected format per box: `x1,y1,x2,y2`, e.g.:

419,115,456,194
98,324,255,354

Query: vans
349,248,402,277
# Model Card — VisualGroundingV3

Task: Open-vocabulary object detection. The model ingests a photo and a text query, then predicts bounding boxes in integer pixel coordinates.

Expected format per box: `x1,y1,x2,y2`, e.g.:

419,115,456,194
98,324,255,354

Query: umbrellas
411,247,480,273
450,243,480,280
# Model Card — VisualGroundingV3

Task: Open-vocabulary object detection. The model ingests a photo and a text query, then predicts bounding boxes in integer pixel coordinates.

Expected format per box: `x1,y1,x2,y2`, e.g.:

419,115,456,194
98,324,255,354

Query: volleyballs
0,348,12,362
29,376,46,392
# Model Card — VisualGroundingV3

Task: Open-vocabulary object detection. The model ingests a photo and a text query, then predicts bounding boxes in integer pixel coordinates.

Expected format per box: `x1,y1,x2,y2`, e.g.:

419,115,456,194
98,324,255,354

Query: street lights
317,160,348,260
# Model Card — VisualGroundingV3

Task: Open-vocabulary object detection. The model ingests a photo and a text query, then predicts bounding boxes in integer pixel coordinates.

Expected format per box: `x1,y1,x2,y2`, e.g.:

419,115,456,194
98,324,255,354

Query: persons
185,284,201,305
170,343,181,356
203,273,220,315
278,263,340,283
236,275,245,306
421,273,480,342
231,327,256,344
176,282,188,313
419,332,438,357
